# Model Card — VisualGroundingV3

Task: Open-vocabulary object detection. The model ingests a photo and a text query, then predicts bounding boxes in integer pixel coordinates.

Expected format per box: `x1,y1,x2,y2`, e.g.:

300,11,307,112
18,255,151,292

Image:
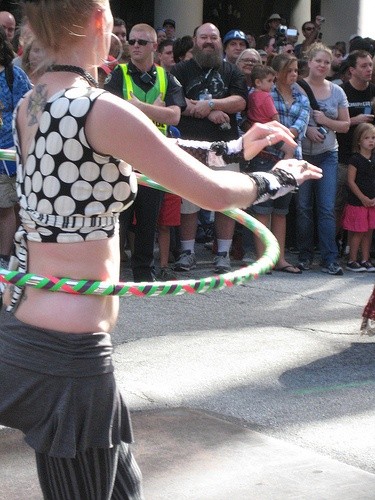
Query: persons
0,7,375,276
1,0,324,500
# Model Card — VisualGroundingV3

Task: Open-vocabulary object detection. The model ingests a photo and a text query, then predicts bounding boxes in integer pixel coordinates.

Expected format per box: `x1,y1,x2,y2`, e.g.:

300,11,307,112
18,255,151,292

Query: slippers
280,265,302,273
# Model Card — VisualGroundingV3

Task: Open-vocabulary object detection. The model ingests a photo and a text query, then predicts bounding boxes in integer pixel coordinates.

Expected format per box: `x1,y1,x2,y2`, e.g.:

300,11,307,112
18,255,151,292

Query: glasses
115,32,125,37
128,39,148,45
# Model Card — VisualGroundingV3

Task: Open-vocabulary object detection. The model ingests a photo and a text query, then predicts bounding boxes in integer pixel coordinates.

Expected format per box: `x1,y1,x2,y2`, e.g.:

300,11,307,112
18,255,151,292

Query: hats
264,13,286,29
163,19,175,27
223,29,249,48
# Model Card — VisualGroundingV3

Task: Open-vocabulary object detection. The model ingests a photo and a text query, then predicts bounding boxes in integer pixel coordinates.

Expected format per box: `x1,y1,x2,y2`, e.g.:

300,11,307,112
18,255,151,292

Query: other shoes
133,267,153,282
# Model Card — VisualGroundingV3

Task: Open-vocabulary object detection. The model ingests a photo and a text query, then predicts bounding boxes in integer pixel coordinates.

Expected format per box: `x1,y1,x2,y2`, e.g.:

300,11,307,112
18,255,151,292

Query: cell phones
319,126,327,136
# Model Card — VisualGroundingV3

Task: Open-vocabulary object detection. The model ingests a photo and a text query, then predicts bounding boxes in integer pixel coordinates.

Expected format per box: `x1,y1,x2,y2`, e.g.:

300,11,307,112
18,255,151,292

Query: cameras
220,121,232,132
274,24,299,44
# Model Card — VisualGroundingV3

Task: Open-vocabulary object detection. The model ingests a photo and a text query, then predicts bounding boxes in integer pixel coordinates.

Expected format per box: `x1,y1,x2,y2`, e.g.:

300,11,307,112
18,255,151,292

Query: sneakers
321,263,344,275
298,261,314,269
345,261,365,271
214,256,231,273
360,261,375,272
175,253,196,271
